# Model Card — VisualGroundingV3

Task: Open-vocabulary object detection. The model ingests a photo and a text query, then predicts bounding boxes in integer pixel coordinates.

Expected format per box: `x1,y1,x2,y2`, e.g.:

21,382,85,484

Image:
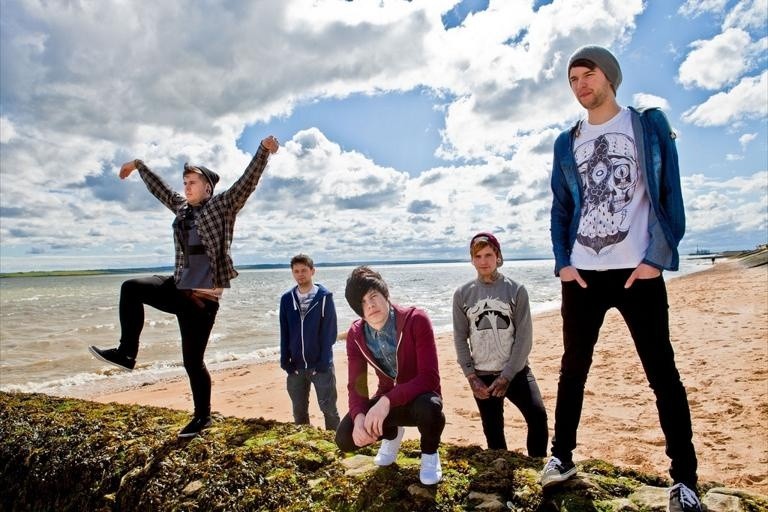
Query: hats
470,232,504,269
566,45,623,97
193,166,220,198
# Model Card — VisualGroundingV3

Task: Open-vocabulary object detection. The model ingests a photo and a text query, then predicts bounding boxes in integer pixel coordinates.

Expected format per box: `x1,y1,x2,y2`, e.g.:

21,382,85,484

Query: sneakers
178,412,213,437
419,450,443,487
88,343,137,373
371,426,406,467
668,480,704,512
539,453,578,489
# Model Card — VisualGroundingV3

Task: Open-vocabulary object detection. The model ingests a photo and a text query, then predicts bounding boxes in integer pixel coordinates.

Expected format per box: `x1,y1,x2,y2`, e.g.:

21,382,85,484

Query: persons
278,253,341,432
334,266,446,486
539,44,705,512
87,135,280,439
450,232,550,458
